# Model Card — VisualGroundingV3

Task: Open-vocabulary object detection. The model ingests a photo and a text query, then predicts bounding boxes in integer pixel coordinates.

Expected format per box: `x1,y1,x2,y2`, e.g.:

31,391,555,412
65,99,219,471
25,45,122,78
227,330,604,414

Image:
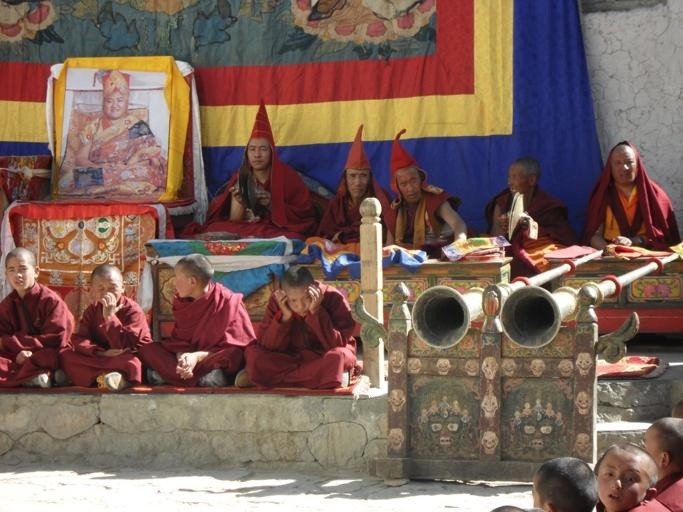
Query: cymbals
233,165,263,210
507,191,530,244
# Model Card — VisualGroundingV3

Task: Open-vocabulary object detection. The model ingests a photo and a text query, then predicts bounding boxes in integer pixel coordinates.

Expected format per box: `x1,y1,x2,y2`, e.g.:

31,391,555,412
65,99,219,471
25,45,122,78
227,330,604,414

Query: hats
336,123,398,238
389,129,444,210
92,68,132,102
244,96,290,229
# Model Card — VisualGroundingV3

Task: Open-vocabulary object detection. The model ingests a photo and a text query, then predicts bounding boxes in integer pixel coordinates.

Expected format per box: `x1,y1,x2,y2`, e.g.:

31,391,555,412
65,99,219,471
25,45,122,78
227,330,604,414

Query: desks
142,238,304,344
306,256,512,304
547,255,683,335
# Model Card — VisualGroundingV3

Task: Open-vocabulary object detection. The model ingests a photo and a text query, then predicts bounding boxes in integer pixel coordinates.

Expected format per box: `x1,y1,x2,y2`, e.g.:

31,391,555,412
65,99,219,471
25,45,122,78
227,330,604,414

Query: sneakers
198,369,227,388
145,366,165,385
95,371,132,392
234,367,256,388
53,369,71,385
31,372,52,389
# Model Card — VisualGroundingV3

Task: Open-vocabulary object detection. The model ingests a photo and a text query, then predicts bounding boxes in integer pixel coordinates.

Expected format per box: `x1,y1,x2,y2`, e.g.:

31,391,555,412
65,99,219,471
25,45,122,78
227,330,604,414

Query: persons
671,400,683,416
385,130,469,252
236,267,361,390
316,123,396,244
205,95,313,234
0,247,75,387
641,416,683,512
484,156,573,245
595,444,672,512
138,255,254,385
585,141,680,250
531,456,599,512
491,505,528,512
61,266,151,388
62,69,168,195
